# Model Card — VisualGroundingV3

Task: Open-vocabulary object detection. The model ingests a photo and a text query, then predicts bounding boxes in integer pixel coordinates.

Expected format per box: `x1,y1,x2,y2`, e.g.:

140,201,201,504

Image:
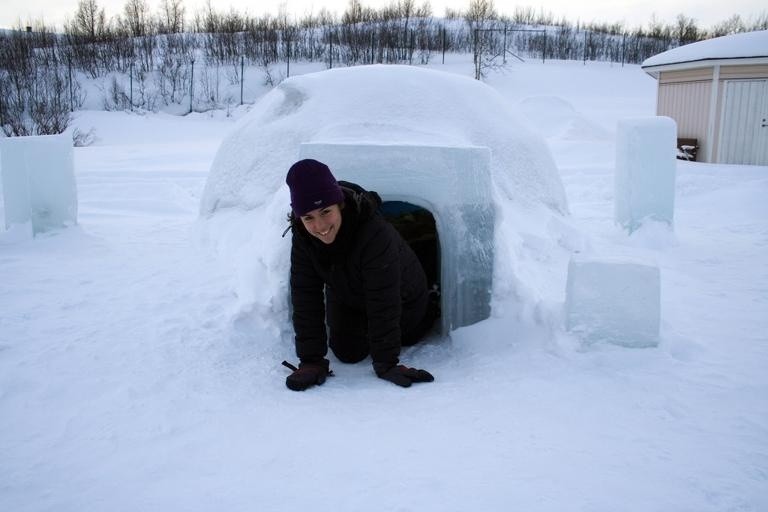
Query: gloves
379,362,435,386
284,357,329,391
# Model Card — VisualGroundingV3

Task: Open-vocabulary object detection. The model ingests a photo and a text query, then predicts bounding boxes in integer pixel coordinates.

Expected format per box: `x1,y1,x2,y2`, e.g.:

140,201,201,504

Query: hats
285,157,346,220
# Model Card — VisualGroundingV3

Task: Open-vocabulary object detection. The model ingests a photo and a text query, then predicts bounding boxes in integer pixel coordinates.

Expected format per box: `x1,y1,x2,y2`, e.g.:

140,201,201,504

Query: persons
281,158,438,390
